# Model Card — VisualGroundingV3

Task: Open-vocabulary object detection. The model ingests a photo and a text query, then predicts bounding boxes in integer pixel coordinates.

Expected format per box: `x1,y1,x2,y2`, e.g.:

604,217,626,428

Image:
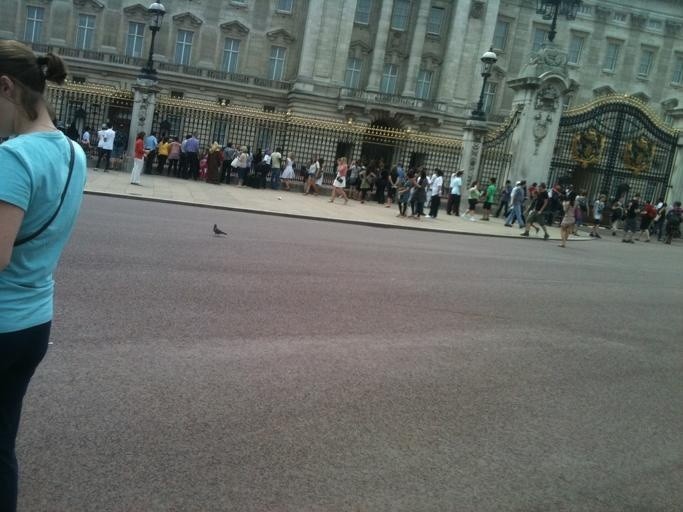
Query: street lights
135,0,167,80
468,46,498,122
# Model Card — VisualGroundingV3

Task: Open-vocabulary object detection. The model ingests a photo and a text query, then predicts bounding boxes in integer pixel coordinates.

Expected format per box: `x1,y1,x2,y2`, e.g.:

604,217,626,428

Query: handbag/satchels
315,169,322,178
575,208,582,221
231,157,240,168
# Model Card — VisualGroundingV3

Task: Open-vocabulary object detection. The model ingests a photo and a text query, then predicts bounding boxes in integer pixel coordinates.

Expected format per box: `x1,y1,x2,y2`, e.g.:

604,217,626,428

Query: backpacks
648,206,658,218
578,198,586,211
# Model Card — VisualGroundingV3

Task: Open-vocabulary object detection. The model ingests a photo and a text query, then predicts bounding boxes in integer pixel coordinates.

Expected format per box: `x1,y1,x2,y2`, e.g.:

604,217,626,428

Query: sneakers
396,211,671,248
93,167,393,207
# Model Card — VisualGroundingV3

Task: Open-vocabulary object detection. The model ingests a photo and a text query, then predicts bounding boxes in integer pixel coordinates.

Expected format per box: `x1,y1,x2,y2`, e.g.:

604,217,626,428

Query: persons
130,131,324,196
330,158,497,222
0,37,87,509
57,120,127,172
494,181,683,247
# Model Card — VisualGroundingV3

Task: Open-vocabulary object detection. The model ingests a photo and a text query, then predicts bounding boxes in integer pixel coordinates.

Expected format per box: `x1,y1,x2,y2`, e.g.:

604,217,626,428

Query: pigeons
213,223,228,237
